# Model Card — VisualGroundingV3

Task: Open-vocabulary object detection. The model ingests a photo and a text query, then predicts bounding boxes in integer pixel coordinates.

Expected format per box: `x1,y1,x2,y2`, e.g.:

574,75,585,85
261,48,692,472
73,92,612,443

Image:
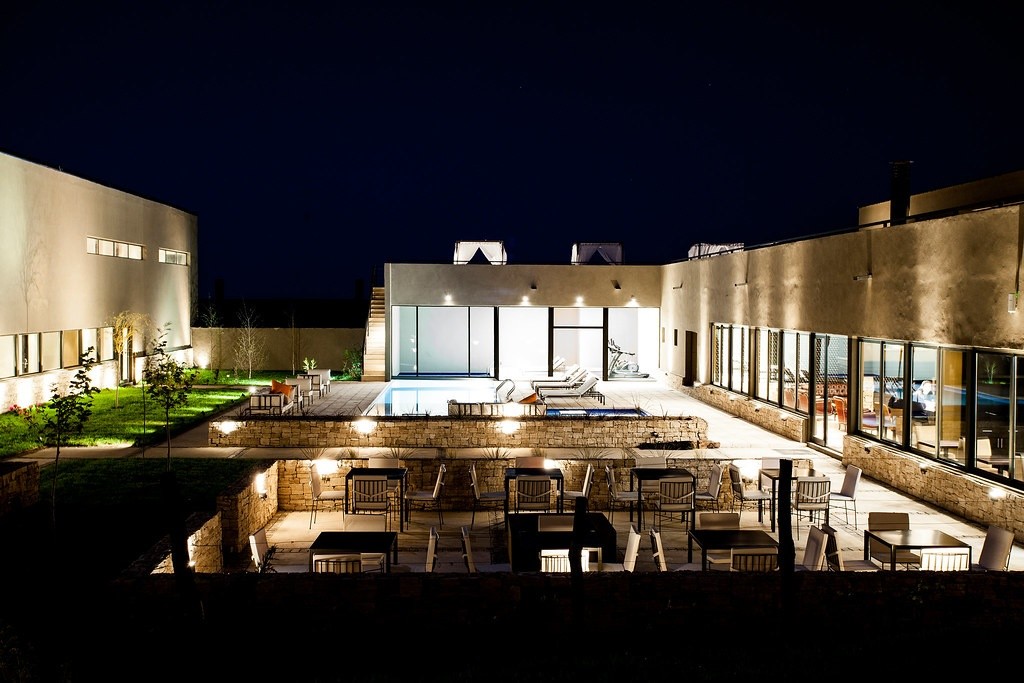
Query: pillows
271,380,290,396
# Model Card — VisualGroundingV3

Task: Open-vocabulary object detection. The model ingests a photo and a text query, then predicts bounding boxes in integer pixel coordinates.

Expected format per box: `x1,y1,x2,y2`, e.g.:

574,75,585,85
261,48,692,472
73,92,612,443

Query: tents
687,243,744,261
570,243,622,265
453,240,508,266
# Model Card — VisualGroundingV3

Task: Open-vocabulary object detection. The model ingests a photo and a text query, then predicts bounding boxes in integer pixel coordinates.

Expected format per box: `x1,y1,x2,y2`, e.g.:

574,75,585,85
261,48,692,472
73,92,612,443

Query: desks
862,417,895,438
503,467,564,513
342,467,410,530
309,531,398,573
629,469,697,530
920,439,958,459
863,529,973,573
977,455,1011,474
758,466,827,532
688,529,779,574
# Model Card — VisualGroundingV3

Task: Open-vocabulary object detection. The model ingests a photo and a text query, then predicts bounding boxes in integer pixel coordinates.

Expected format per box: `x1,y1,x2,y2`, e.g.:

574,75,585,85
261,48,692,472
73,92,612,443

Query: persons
888,381,935,423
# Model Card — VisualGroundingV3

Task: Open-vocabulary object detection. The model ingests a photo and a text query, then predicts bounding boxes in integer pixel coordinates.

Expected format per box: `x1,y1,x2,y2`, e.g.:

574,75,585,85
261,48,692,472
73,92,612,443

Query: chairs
247,355,1016,574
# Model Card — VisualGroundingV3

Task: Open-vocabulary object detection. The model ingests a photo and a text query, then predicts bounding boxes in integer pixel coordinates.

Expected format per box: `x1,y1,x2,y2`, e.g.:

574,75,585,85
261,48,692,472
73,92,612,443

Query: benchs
249,394,285,416
297,374,325,398
815,382,849,397
285,378,314,405
307,369,332,394
269,384,303,412
